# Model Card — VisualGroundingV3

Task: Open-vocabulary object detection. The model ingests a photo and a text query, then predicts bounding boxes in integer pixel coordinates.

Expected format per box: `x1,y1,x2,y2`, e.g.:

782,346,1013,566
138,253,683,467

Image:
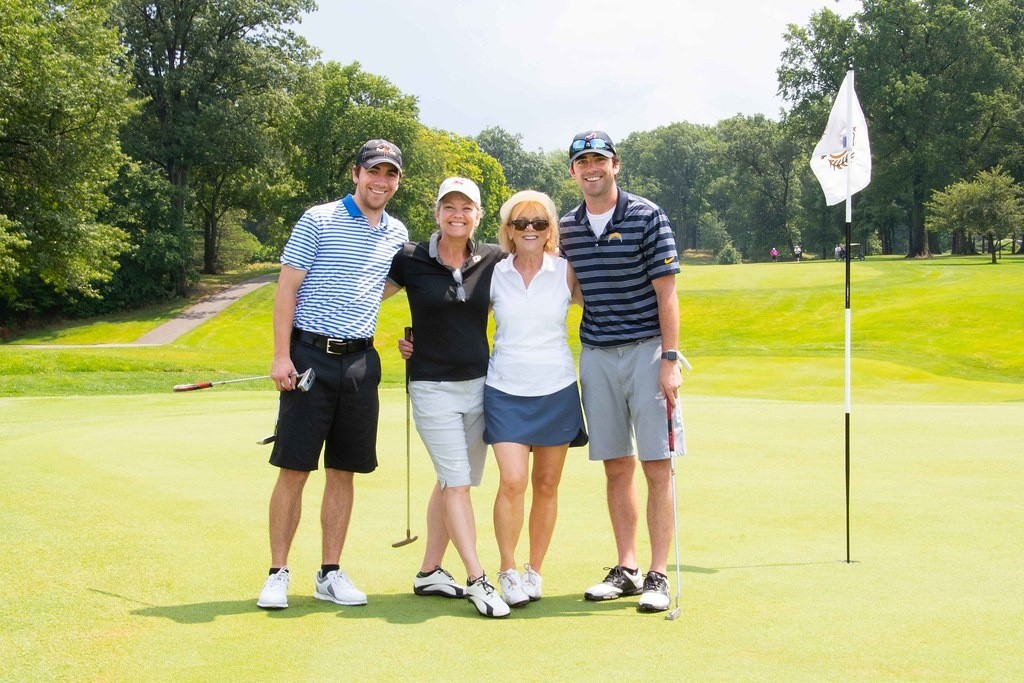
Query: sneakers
414,565,467,598
638,571,670,611
468,573,511,617
521,563,542,600
256,565,289,608
314,569,368,605
583,566,643,600
498,569,530,607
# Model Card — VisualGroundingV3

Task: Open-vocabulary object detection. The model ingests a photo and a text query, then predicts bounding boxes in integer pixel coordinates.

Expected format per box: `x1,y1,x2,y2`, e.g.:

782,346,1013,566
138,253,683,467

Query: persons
770,248,778,262
382,176,511,617
835,243,845,261
794,246,801,261
257,138,410,609
398,190,584,608
558,131,683,610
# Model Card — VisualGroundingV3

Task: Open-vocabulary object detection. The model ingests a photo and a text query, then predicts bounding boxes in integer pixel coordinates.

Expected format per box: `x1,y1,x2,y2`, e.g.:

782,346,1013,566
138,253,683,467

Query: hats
355,138,402,175
438,177,480,205
568,131,617,164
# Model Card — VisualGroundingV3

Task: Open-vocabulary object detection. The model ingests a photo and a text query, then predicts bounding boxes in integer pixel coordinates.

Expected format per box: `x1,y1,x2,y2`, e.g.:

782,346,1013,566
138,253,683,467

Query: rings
290,372,298,376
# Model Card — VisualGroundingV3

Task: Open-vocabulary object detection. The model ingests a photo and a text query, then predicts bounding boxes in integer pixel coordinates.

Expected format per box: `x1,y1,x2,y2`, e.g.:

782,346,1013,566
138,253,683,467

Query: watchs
661,350,678,361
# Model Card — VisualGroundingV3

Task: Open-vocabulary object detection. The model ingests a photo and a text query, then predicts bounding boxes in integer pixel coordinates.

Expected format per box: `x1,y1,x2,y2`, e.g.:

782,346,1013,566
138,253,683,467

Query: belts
291,328,374,355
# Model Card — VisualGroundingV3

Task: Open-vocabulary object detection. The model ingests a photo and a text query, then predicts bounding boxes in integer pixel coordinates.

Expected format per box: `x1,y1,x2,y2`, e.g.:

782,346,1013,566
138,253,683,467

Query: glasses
570,139,609,151
507,220,550,231
453,269,466,302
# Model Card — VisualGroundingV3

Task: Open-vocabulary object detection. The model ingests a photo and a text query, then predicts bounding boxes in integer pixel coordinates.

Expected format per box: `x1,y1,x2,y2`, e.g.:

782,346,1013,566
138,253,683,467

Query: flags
809,70,872,206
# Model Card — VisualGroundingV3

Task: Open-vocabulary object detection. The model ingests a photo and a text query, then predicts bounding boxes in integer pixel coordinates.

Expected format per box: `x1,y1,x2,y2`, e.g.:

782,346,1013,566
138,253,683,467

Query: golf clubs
664,391,682,621
390,324,419,549
172,367,317,394
